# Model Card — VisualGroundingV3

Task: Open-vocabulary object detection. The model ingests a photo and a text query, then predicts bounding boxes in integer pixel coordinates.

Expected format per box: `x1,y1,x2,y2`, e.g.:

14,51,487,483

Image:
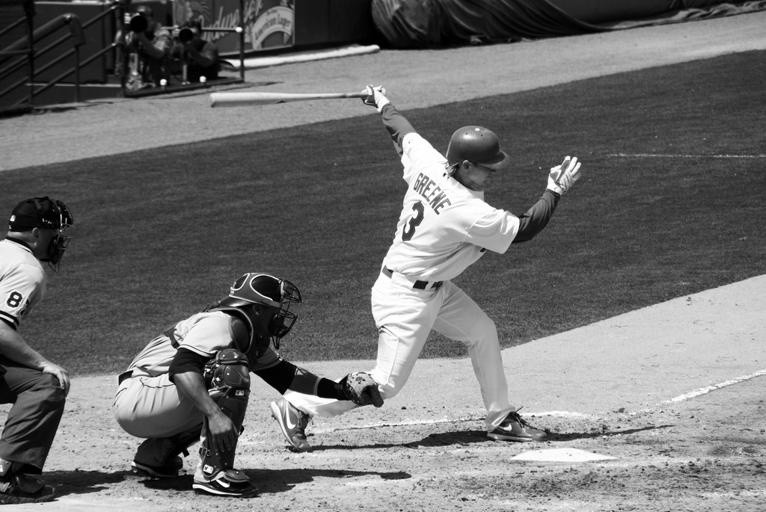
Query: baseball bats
210,87,386,107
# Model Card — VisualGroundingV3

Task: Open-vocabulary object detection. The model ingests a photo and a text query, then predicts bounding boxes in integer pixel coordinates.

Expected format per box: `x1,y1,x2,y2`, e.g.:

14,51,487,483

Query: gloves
359,82,393,116
546,153,584,195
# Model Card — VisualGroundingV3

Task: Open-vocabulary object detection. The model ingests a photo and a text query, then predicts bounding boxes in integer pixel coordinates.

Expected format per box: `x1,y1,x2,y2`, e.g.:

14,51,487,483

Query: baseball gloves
347,371,384,407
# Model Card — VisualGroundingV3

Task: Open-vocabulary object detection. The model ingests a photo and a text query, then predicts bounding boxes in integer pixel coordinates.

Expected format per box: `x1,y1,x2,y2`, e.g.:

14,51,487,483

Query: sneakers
270,395,313,452
0,458,59,503
484,412,548,445
192,459,262,498
132,455,187,483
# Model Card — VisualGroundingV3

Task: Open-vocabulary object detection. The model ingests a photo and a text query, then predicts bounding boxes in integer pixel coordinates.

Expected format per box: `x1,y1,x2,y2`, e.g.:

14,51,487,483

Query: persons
0,196,73,503
270,85,584,452
163,21,220,84
111,272,383,497
115,6,172,92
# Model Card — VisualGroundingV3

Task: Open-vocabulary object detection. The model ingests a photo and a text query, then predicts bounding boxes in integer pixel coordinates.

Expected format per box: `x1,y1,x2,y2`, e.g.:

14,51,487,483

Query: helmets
8,193,75,236
221,271,299,340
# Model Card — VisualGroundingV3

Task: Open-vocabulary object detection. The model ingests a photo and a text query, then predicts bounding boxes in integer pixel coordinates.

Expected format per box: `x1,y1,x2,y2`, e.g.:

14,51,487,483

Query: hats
445,125,511,171
